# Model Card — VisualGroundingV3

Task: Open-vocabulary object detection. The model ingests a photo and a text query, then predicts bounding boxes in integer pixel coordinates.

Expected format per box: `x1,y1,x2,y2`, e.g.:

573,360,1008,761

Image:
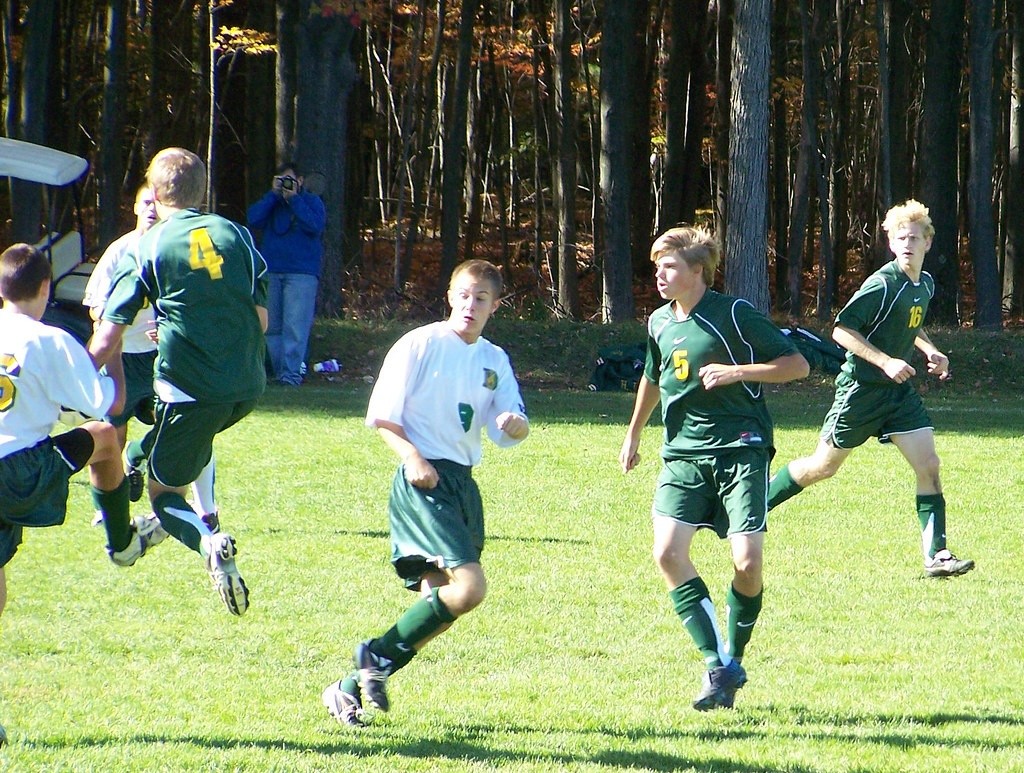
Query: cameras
277,175,298,190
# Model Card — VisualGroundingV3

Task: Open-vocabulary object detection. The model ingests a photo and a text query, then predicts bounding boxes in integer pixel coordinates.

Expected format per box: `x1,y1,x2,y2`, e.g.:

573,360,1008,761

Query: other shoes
275,380,291,386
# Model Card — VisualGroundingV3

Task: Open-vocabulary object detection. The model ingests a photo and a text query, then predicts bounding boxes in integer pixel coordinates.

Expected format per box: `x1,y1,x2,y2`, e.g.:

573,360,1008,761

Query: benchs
41,230,98,303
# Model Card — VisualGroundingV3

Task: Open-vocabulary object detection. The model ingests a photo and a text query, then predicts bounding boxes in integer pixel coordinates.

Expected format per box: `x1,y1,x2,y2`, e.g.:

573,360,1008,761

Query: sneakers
104,512,170,567
187,499,220,534
924,549,975,578
90,510,104,527
693,660,747,711
205,533,250,617
321,678,365,730
351,637,393,713
121,440,146,503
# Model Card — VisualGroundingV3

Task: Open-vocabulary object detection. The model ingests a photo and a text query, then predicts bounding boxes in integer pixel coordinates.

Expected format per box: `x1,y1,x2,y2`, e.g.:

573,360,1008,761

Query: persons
87,147,270,617
81,183,160,528
246,162,325,385
768,199,975,578
618,228,811,712
0,242,170,748
322,260,529,730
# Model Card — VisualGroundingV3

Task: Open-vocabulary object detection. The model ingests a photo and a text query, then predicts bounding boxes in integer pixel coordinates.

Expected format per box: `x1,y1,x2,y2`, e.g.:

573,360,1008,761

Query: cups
313,359,342,372
